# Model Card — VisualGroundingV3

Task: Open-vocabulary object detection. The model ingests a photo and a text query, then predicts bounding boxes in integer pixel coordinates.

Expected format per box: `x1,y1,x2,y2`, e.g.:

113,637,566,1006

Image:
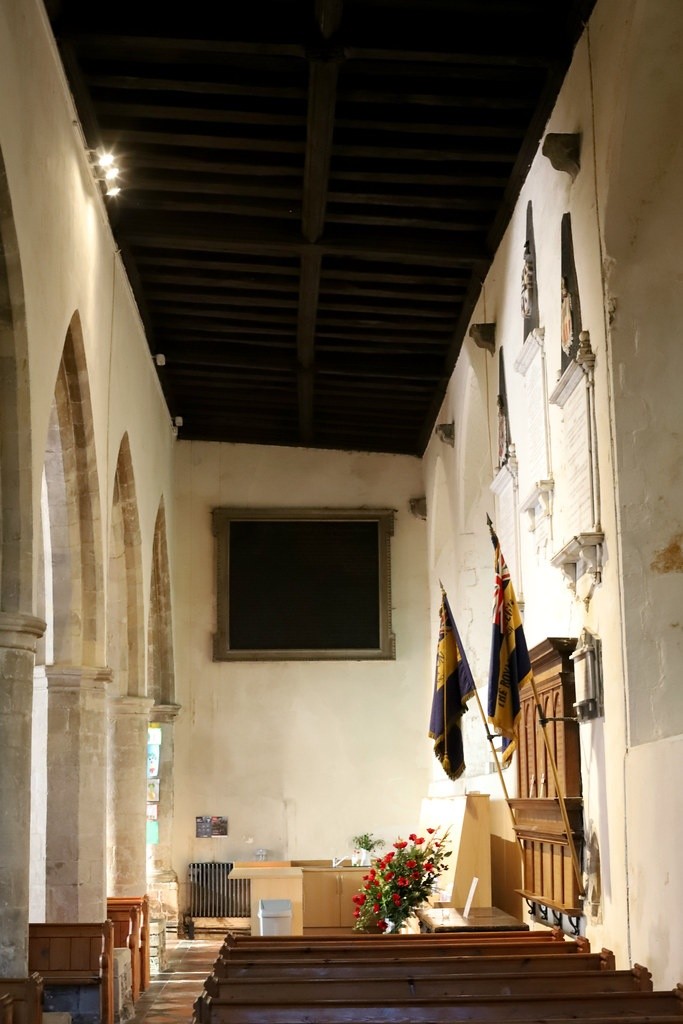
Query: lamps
172,415,183,426
410,496,428,519
542,132,580,178
435,423,455,446
85,144,120,196
151,354,165,365
469,322,496,353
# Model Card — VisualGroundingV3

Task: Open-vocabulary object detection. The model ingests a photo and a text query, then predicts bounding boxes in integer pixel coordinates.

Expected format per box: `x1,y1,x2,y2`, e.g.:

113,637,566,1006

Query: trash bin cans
258,899,292,935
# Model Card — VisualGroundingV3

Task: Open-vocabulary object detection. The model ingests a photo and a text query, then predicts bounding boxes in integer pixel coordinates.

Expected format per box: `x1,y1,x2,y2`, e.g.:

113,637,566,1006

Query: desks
413,908,530,932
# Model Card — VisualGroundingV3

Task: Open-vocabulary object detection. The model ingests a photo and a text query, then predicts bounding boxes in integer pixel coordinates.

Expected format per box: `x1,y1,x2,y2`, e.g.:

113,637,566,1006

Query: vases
398,912,420,934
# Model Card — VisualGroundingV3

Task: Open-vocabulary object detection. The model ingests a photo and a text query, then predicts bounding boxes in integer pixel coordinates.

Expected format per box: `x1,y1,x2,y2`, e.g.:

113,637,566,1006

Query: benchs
187,924,683,1024
0,895,150,1024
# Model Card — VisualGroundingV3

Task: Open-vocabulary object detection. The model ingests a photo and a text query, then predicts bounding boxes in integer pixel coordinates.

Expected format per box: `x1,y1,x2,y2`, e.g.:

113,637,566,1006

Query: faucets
332,855,346,868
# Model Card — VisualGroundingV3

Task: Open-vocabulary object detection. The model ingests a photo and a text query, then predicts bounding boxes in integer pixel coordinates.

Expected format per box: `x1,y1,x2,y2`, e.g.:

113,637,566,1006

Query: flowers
352,822,455,932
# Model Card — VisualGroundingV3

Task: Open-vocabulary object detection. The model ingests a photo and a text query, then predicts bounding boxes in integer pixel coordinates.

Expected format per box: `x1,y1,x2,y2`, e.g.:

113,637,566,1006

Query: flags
487,542,533,769
428,595,477,782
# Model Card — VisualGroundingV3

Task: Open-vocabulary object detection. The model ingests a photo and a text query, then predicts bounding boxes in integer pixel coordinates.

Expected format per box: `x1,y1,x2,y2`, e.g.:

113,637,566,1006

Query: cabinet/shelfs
302,871,385,927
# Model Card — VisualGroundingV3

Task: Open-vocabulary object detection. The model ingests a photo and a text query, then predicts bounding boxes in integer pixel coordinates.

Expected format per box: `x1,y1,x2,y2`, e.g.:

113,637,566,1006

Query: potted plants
353,833,385,868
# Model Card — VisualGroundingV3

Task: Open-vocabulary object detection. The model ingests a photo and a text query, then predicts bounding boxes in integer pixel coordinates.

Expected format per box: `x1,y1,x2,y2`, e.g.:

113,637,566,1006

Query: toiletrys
351,850,360,867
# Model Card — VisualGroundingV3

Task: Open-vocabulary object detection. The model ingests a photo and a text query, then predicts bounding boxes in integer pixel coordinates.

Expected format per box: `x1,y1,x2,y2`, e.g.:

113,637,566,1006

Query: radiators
187,863,252,919
